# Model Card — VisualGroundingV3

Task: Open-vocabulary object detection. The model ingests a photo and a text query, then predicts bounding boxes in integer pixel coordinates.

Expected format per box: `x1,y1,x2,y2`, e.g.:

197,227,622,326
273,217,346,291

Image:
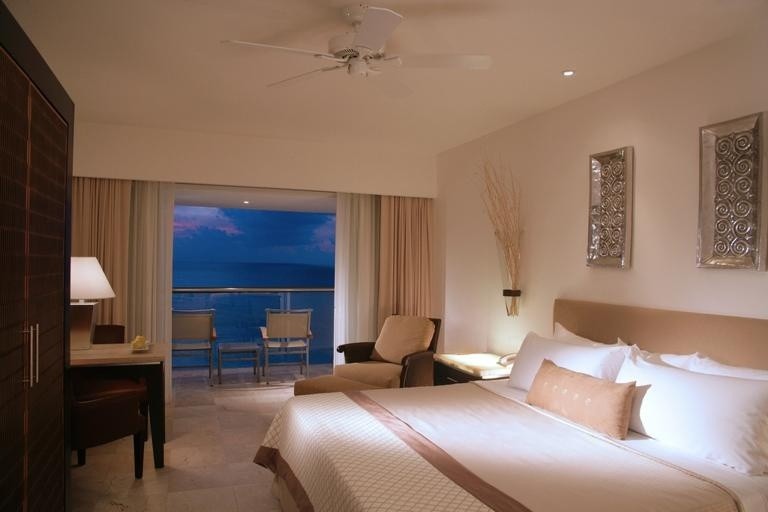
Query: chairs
260,308,313,384
335,315,442,389
170,309,217,387
92,325,148,441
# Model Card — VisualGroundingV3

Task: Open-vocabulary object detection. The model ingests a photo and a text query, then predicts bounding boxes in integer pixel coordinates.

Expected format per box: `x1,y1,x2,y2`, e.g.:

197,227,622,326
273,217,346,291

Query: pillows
512,323,768,474
372,315,436,363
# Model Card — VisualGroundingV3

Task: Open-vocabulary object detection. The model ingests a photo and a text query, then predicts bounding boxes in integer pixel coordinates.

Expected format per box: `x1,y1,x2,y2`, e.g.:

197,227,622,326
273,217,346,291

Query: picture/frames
586,147,635,270
697,111,768,272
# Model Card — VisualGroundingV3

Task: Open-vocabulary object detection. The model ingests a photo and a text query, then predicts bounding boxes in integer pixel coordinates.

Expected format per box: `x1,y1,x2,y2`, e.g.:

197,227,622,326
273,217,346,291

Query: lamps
67,254,116,352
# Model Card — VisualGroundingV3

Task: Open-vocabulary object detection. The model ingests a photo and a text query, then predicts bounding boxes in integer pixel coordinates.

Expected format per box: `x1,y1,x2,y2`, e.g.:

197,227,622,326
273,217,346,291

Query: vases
503,237,525,316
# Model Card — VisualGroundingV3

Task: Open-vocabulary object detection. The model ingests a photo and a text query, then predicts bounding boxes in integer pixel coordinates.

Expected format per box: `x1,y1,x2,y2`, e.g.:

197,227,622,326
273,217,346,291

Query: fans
222,0,494,90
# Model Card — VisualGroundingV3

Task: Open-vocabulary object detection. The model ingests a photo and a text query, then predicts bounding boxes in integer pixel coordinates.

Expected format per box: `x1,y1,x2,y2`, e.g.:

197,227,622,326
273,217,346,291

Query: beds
287,297,768,512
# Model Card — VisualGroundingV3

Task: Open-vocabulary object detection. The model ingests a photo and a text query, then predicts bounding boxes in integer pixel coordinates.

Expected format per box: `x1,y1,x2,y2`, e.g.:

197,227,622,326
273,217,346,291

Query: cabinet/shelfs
0,0,73,512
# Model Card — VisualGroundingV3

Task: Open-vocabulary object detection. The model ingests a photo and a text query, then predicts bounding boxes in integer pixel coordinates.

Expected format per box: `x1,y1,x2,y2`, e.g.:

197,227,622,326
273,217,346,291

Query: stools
293,374,379,393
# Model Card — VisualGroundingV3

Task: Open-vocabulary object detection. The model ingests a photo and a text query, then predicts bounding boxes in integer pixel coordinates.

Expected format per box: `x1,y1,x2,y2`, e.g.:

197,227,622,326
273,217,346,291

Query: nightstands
431,352,514,386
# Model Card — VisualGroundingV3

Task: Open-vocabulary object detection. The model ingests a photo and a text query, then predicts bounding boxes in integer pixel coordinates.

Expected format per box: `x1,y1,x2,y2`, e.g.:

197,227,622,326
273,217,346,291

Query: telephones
499,353,518,366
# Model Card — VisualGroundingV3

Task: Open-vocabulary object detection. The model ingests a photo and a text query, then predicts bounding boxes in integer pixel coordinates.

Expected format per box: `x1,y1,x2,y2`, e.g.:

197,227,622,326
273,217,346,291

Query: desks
67,342,166,469
217,343,261,384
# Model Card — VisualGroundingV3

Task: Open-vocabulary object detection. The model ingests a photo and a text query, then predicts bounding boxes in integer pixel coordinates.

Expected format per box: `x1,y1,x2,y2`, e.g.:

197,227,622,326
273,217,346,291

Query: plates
130,344,148,351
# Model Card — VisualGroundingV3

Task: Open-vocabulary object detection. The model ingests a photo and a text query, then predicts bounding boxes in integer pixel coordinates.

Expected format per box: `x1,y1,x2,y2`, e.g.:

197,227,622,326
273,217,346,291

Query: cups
133,335,146,348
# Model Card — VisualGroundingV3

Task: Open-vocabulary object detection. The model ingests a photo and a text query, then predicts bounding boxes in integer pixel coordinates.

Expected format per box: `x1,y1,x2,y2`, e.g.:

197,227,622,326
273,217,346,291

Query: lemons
132,336,146,349
